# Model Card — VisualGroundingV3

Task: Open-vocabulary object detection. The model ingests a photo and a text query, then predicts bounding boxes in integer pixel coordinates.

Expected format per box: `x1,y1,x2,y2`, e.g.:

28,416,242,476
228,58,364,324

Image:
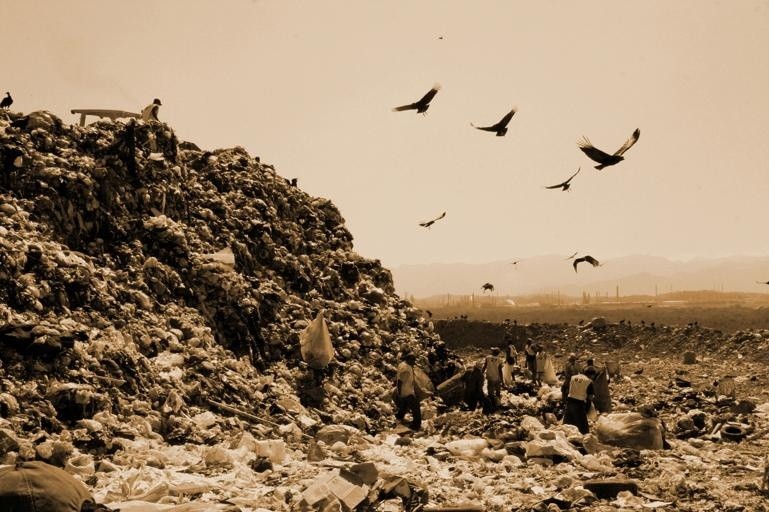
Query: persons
561,352,579,407
536,343,548,387
482,346,505,399
141,99,162,123
503,338,518,381
397,353,421,432
523,337,538,386
562,366,598,435
582,358,599,413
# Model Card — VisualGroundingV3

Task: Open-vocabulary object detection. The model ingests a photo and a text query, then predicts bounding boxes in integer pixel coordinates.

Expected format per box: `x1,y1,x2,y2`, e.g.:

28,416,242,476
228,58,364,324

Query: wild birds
713,378,718,386
634,367,643,375
751,375,756,381
576,128,640,170
0,92,13,110
566,253,577,259
391,84,441,117
544,169,580,191
419,212,445,227
471,109,517,136
574,256,598,272
481,283,494,292
512,261,519,265
